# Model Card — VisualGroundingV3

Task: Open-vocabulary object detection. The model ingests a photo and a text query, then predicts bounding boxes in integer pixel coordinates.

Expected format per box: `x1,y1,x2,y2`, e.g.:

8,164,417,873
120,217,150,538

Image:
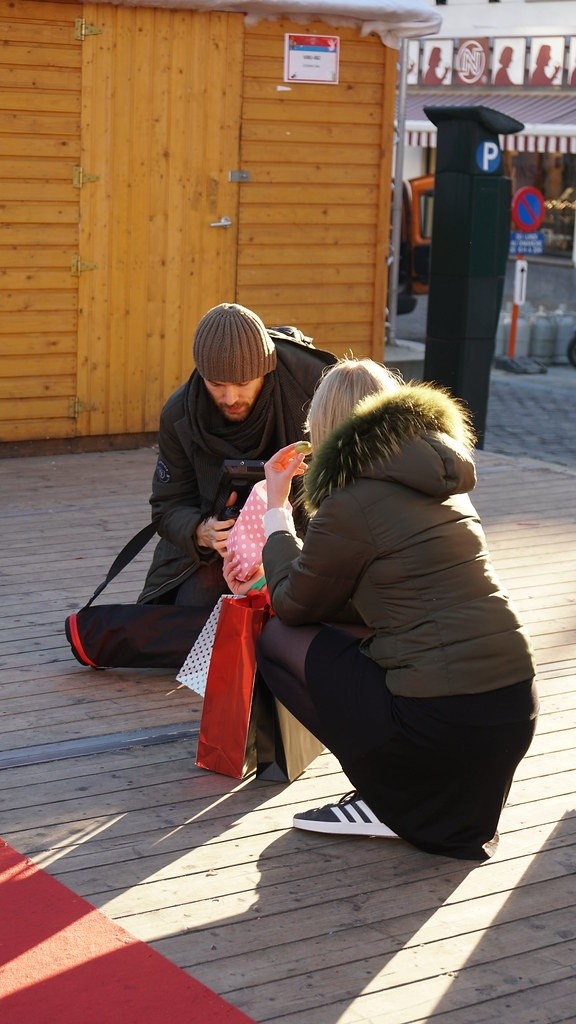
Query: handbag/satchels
62,493,218,671
190,586,275,780
172,590,247,704
266,690,328,786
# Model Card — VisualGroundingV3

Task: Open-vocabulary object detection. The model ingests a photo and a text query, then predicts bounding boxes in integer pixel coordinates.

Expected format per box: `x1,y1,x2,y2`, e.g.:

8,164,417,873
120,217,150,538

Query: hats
190,303,279,384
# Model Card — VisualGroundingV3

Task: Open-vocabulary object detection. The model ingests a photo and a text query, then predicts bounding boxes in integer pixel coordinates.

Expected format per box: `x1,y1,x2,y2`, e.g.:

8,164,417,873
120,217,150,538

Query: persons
255,353,540,862
133,305,344,607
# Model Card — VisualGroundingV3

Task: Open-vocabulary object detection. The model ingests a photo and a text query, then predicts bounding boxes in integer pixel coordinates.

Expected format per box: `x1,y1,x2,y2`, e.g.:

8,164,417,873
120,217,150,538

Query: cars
384,174,435,314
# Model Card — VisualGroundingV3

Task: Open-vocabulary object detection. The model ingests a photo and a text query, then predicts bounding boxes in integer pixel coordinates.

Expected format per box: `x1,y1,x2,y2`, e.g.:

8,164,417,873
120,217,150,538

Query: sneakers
289,791,403,841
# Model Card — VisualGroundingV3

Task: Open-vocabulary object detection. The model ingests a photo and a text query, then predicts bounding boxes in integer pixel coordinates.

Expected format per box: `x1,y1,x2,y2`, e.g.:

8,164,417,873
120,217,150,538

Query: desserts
295,442,313,457
